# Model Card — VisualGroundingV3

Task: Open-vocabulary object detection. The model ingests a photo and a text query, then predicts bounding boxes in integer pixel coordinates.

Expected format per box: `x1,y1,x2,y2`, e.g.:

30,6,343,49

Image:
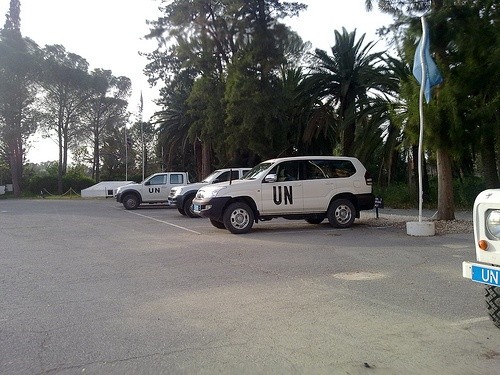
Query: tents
81,181,136,197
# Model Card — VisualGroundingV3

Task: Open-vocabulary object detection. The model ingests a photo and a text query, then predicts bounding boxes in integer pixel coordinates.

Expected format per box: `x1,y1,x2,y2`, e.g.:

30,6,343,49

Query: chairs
279,169,288,181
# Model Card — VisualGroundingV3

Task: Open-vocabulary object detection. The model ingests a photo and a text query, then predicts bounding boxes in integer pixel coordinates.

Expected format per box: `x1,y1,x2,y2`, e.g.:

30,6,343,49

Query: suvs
193,156,376,234
168,168,254,219
461,188,500,328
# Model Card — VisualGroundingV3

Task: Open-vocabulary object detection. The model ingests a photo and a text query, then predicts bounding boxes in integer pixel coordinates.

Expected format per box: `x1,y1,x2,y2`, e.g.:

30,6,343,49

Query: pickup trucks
115,171,193,210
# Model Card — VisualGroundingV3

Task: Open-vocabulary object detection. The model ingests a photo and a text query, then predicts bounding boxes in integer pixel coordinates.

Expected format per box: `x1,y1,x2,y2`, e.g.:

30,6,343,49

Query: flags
412,25,442,104
